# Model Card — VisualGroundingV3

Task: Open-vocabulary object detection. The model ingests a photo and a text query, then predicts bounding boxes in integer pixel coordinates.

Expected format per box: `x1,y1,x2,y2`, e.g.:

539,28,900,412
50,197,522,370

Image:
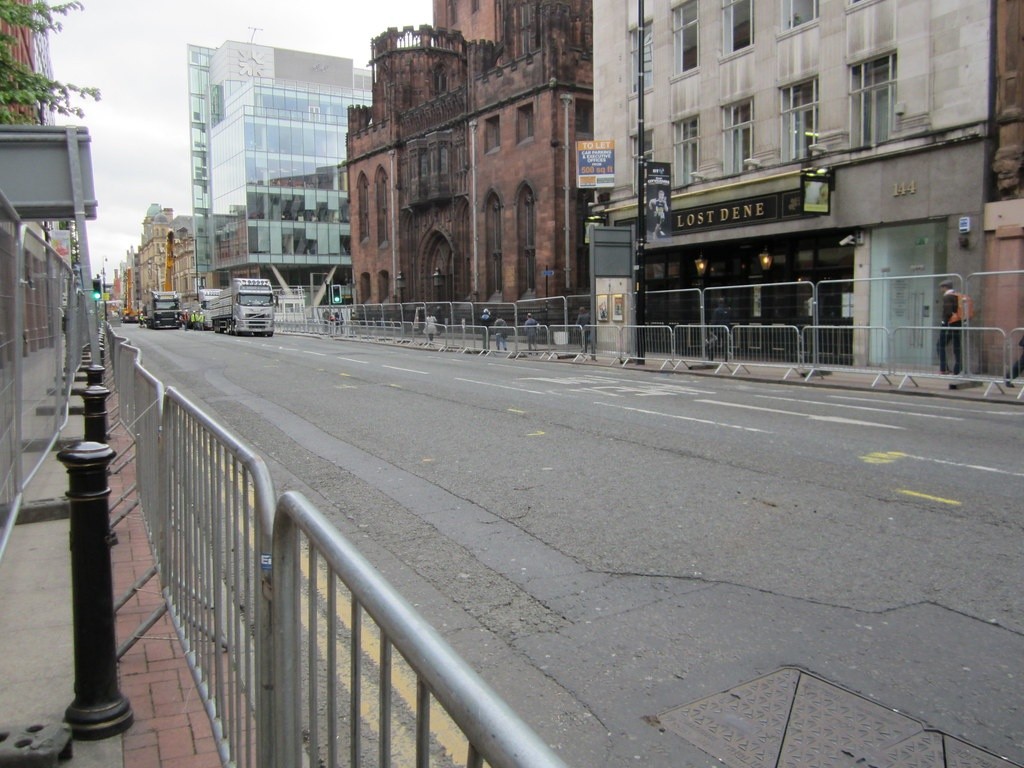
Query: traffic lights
92,278,102,300
331,284,342,304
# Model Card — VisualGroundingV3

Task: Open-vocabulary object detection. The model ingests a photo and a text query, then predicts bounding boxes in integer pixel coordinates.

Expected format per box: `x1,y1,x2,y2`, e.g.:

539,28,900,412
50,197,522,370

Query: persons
481,308,493,349
1004,336,1024,388
649,191,669,240
335,311,344,335
138,311,144,327
936,280,965,375
494,315,507,351
182,310,190,331
524,312,539,354
574,307,590,353
322,309,334,332
423,313,437,342
191,311,205,330
708,298,732,361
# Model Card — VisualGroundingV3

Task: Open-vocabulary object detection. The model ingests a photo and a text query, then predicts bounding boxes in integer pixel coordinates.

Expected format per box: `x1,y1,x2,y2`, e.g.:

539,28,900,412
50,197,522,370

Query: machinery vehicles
121,266,144,323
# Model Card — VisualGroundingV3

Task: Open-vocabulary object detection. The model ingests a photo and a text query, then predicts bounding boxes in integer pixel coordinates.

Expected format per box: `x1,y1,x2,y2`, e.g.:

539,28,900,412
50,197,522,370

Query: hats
939,279,953,288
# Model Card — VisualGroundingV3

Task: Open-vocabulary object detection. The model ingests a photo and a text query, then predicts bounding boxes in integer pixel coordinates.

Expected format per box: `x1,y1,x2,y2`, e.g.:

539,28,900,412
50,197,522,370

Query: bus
142,290,182,331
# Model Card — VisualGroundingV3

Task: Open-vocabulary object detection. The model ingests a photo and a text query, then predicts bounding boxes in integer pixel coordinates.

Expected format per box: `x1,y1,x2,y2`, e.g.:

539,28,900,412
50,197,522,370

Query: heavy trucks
179,288,222,330
207,278,276,337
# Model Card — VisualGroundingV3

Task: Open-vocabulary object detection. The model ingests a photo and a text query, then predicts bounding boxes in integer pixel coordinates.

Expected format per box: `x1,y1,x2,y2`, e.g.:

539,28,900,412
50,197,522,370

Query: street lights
103,254,108,322
467,120,480,302
387,150,398,304
558,92,576,297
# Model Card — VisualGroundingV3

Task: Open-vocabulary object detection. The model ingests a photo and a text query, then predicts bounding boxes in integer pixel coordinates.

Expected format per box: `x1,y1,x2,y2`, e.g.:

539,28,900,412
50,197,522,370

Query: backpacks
945,292,972,324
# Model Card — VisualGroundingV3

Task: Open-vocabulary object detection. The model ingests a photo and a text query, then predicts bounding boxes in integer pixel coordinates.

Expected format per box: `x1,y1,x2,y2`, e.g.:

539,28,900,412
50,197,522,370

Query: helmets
193,311,196,313
185,310,188,312
200,312,203,314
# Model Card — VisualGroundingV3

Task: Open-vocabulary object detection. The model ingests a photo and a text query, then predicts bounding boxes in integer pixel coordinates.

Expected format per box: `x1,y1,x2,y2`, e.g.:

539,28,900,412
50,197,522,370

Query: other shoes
936,369,949,373
1005,376,1014,387
